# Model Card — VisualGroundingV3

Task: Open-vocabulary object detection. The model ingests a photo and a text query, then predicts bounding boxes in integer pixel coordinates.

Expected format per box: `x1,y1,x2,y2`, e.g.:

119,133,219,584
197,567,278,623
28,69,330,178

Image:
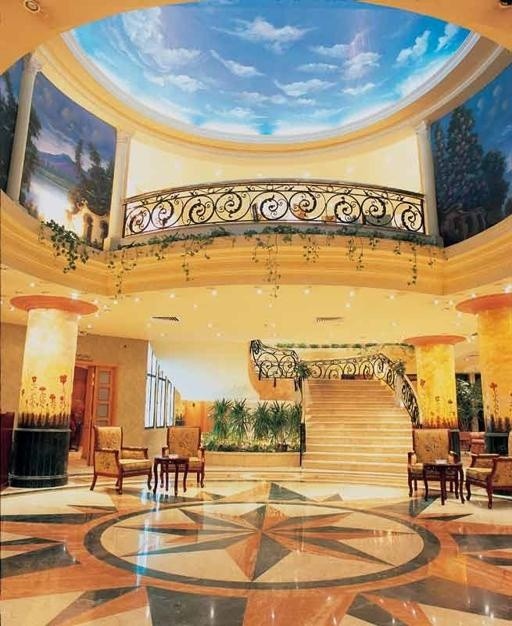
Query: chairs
407,429,459,496
90,425,152,494
465,430,512,509
162,427,205,488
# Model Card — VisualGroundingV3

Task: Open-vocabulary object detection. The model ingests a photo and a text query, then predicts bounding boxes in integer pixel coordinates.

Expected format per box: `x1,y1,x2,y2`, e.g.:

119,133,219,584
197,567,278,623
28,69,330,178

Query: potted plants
254,401,303,451
